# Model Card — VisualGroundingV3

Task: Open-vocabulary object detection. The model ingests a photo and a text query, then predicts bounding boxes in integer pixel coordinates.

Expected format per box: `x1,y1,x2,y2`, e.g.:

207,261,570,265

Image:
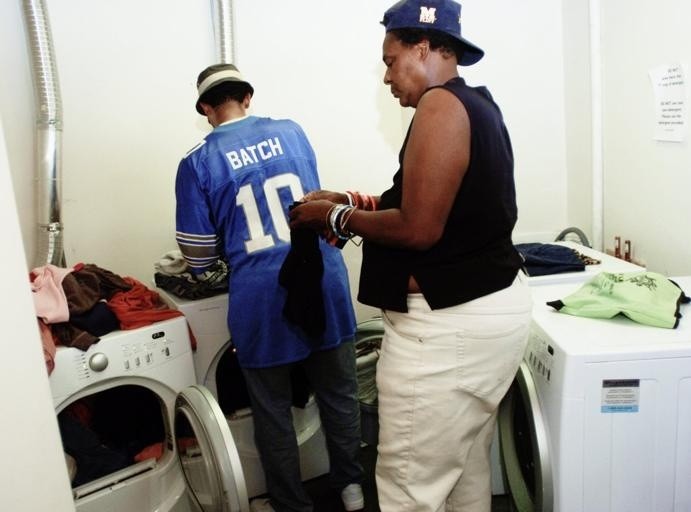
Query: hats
380,1,485,67
196,63,255,117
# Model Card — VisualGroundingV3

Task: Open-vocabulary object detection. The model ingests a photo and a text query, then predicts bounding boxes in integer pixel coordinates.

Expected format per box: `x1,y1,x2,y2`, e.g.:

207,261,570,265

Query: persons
280,1,536,512
173,63,369,511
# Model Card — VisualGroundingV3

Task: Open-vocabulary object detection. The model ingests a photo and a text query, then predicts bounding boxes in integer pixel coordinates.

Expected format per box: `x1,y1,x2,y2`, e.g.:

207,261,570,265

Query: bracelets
327,191,377,245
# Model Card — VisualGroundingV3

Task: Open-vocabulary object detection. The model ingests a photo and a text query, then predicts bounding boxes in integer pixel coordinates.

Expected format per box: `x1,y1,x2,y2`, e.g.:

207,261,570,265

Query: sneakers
337,483,366,511
248,498,274,511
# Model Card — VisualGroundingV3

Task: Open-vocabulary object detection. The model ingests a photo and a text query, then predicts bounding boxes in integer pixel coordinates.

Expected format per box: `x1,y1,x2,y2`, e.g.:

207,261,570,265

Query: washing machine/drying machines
40,317,251,512
155,255,330,498
344,257,386,450
501,280,691,511
